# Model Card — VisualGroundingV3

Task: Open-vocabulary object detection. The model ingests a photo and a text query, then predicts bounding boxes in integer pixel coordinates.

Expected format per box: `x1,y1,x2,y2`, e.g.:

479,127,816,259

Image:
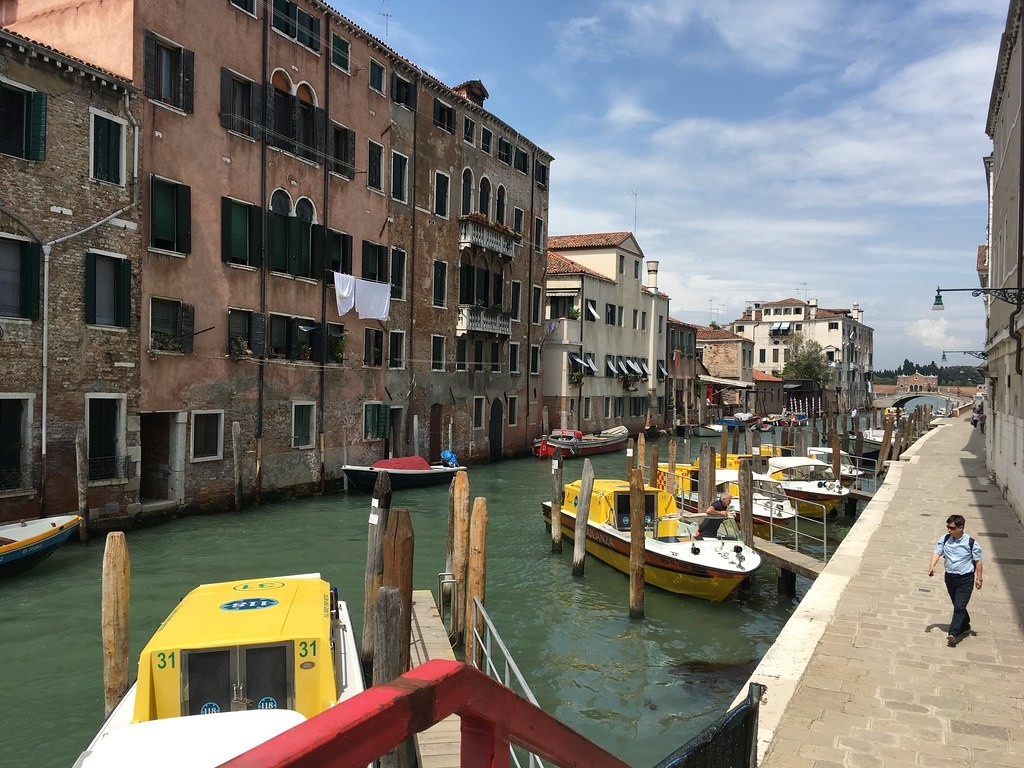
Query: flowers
627,374,639,384
492,221,526,240
467,211,487,225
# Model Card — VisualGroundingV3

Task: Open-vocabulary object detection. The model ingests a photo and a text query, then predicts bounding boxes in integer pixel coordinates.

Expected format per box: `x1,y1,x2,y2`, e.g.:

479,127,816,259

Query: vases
492,226,503,233
513,234,521,240
469,217,484,225
503,230,513,237
628,376,638,382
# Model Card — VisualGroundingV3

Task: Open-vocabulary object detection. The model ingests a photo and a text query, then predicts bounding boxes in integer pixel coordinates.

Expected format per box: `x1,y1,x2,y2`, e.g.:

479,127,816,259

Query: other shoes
947,633,956,647
960,631,968,634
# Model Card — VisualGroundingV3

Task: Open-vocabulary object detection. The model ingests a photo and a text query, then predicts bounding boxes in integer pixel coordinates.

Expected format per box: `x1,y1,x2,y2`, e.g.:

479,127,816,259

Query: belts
947,573,972,578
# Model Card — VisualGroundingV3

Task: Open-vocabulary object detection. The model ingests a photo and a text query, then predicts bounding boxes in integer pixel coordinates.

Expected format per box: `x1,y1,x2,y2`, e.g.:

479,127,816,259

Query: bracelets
976,578,983,582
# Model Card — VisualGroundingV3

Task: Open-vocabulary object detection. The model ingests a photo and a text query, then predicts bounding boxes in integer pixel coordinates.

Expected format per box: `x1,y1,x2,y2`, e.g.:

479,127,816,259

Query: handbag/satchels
971,418,974,425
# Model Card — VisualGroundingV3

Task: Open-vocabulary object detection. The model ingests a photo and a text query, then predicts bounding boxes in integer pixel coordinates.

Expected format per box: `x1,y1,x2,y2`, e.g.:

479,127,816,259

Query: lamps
941,350,987,362
932,286,1024,310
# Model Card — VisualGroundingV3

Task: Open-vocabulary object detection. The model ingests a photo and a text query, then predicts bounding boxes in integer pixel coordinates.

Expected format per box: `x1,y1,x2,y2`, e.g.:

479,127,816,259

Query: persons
970,398,986,434
927,383,962,397
851,405,857,432
696,494,733,539
928,515,984,646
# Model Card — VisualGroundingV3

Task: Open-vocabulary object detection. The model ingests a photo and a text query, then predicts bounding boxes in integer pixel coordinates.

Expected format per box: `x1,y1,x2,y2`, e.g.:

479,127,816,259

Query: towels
333,271,356,317
355,278,391,322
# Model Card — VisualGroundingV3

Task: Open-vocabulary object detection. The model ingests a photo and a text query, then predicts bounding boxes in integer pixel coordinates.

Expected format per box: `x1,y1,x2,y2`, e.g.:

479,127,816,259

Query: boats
653,461,801,540
346,449,471,492
693,453,852,519
532,426,630,461
698,402,911,484
1,515,85,578
542,478,762,602
67,573,380,768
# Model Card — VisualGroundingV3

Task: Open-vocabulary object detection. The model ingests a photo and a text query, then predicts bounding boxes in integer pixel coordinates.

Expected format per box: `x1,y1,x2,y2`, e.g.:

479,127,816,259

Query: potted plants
575,382,584,388
568,307,581,320
642,378,649,383
571,372,585,378
327,332,350,362
681,352,685,358
470,297,488,314
687,401,693,409
659,378,665,383
501,308,513,321
490,302,504,314
627,387,638,392
296,345,313,360
687,352,694,359
668,397,675,410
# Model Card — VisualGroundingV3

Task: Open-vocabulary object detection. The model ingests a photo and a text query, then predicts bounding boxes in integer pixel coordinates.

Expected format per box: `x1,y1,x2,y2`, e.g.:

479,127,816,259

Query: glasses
947,525,959,530
